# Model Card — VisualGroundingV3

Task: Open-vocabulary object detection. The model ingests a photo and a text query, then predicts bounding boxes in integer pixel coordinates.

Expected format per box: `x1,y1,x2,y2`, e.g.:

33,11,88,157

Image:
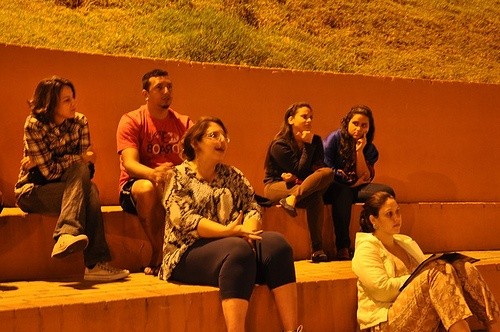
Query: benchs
0,200,500,332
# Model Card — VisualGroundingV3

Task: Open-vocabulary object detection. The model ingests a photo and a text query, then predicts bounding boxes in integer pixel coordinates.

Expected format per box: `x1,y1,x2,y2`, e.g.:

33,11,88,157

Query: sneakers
84,262,130,280
51,233,88,258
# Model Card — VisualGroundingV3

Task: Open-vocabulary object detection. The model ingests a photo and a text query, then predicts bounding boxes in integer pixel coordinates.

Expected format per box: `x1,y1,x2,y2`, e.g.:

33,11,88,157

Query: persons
323,104,395,261
158,116,304,332
352,192,500,332
14,77,130,281
262,102,335,262
116,68,195,274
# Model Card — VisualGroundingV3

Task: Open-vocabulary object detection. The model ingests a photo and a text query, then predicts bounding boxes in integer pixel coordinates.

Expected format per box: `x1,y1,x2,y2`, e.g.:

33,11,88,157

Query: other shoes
312,249,328,261
336,250,352,259
279,198,296,217
144,261,161,275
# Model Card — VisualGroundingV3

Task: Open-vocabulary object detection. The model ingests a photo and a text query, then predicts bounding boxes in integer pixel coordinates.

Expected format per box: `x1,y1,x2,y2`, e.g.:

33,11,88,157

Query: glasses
203,133,230,143
